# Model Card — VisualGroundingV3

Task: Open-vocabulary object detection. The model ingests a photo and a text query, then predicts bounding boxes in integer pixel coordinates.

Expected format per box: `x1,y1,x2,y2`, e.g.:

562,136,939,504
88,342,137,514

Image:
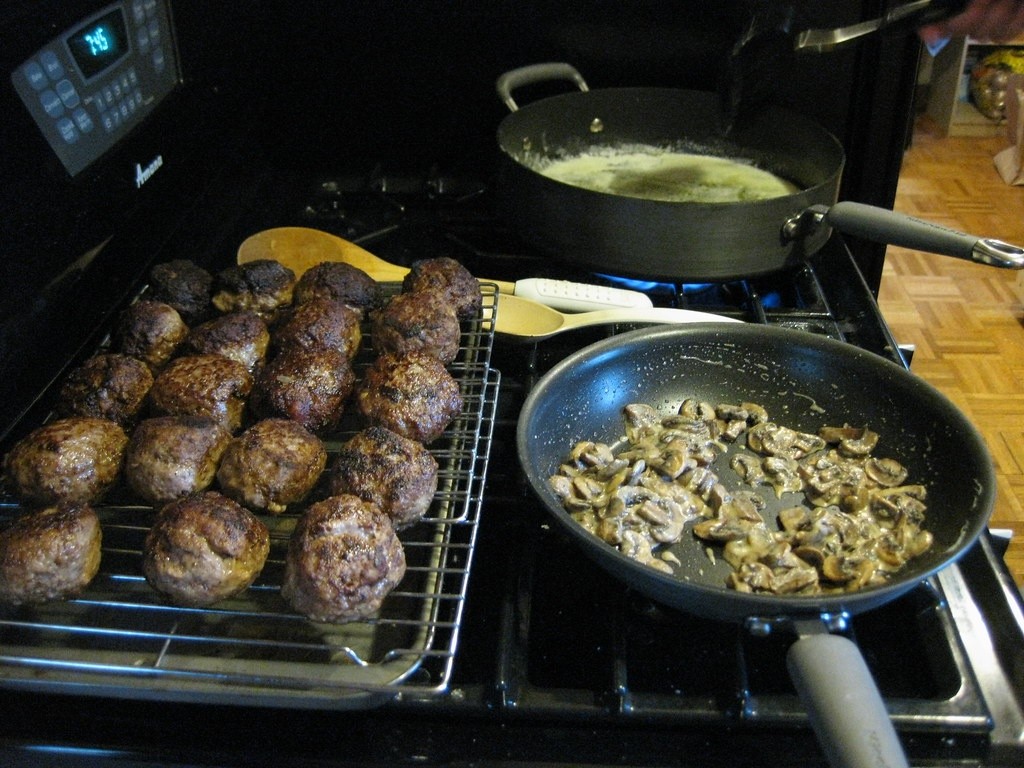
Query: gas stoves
0,158,1024,768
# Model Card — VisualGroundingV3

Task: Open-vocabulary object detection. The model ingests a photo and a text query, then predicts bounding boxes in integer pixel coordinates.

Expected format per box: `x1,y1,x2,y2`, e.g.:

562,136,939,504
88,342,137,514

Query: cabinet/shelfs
918,33,1024,140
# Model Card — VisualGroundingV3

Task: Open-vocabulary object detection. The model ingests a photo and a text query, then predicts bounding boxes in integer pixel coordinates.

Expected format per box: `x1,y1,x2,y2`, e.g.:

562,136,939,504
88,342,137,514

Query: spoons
480,292,745,341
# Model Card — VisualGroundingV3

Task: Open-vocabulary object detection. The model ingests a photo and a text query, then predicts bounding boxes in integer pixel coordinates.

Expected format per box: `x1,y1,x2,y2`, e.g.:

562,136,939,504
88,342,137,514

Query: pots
496,62,1024,278
516,323,995,768
